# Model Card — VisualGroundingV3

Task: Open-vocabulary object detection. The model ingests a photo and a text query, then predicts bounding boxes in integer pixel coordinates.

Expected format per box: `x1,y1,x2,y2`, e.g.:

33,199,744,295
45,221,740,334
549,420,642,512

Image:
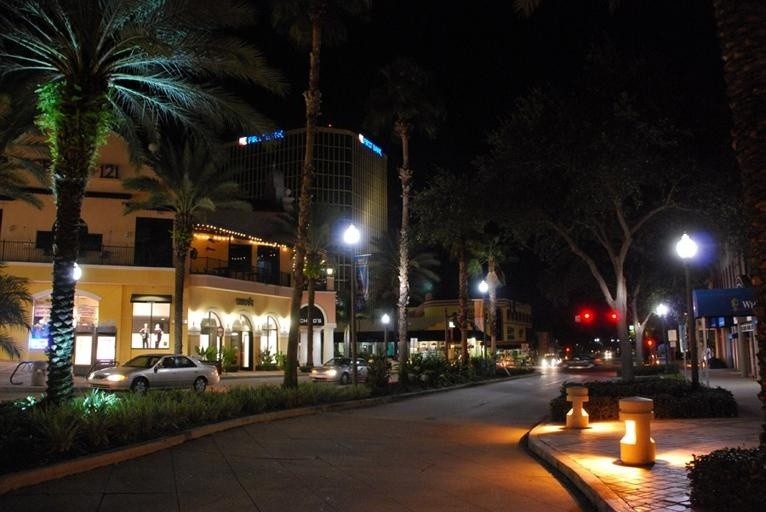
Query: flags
355,253,373,302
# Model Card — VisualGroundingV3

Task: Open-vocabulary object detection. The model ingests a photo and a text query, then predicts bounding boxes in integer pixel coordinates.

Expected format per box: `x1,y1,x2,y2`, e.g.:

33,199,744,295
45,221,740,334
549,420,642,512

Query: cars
540,352,606,372
312,355,372,384
87,351,222,399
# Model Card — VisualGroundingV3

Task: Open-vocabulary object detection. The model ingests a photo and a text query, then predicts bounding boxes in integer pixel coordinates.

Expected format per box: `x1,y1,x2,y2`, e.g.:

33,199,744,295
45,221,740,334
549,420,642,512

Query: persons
705,341,713,368
139,322,150,348
153,323,164,348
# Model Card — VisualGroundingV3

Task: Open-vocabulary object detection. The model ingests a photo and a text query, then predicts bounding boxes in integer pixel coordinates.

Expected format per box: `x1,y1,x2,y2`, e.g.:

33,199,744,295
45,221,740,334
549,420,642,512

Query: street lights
478,278,489,370
382,313,390,368
657,303,672,373
594,336,601,355
610,338,620,357
339,224,361,390
672,228,703,384
